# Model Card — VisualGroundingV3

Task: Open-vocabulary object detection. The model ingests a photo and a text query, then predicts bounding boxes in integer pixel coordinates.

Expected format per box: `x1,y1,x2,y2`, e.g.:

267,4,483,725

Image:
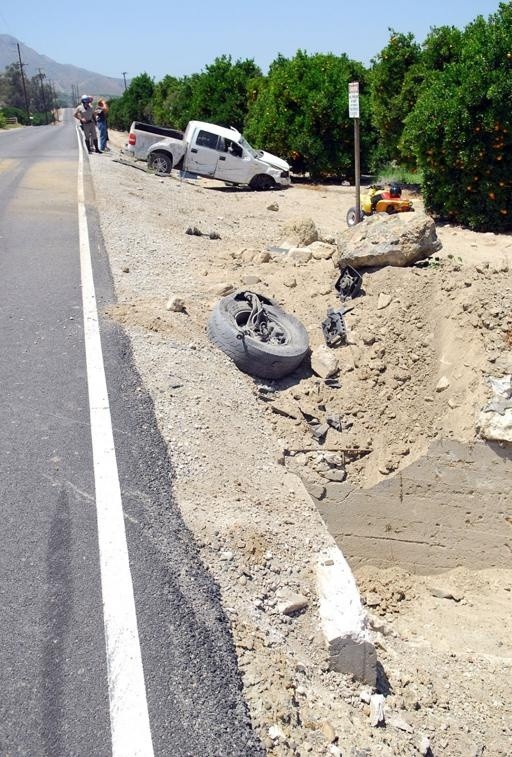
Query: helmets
390,187,401,197
82,96,89,103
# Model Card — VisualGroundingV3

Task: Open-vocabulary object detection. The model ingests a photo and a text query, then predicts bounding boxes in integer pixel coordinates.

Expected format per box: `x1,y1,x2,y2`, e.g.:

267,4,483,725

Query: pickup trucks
122,119,293,192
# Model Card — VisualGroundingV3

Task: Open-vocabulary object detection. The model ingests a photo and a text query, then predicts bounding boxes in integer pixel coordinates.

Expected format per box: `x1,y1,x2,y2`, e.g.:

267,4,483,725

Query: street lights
120,71,128,89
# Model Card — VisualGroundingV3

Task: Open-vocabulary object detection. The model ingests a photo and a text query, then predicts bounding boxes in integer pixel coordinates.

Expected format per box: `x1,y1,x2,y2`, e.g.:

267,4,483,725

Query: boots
93,139,102,153
85,139,91,151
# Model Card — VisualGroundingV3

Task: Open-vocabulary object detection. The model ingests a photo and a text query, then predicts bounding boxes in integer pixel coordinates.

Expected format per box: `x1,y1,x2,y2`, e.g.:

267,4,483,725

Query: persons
73,94,103,154
94,98,111,152
78,95,98,151
369,184,414,213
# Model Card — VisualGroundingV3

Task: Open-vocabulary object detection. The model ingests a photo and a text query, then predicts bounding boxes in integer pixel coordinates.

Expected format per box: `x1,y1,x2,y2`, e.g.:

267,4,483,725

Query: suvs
346,185,415,227
